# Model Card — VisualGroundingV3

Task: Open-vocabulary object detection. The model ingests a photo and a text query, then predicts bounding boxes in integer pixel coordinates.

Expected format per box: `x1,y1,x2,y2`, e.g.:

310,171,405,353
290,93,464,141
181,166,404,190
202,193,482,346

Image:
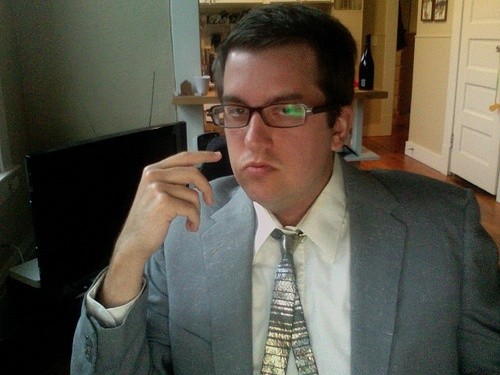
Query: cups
195,74,210,97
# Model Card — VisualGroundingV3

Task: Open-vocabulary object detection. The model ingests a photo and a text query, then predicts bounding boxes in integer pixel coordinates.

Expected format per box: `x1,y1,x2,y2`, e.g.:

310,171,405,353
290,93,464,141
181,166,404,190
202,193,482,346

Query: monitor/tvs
25,121,187,299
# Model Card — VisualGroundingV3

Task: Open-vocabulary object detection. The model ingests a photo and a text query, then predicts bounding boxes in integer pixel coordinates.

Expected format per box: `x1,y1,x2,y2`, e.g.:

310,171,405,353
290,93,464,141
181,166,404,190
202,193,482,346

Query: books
199,42,216,84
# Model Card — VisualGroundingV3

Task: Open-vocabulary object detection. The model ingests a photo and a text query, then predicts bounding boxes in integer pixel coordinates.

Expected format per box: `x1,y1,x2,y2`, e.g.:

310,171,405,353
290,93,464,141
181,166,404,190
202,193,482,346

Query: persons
69,2,500,375
435,0,445,16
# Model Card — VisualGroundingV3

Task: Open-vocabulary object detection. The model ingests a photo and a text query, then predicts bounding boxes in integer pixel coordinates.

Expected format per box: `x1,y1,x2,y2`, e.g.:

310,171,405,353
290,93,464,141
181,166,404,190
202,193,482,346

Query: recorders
191,131,219,152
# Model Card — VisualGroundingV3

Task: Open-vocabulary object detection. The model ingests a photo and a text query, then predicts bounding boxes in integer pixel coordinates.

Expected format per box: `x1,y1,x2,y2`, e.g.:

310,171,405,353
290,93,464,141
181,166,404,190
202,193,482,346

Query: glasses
205,101,339,128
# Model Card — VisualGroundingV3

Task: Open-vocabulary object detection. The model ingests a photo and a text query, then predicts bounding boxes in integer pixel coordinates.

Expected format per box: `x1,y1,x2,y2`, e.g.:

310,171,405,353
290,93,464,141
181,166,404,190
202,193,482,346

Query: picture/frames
433,0,448,22
420,0,432,20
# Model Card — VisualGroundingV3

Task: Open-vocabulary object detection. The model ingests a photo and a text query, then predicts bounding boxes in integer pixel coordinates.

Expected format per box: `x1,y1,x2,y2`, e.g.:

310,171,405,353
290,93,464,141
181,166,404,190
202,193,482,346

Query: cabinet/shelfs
449,0,500,197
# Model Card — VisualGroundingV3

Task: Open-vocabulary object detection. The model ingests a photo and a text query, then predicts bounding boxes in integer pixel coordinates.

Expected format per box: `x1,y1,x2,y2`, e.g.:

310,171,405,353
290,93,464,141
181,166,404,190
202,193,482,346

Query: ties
261,229,319,374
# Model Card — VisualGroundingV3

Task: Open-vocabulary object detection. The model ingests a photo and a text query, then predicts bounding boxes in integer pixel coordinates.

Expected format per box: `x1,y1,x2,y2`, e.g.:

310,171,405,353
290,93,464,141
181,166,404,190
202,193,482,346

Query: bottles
358,34,375,91
